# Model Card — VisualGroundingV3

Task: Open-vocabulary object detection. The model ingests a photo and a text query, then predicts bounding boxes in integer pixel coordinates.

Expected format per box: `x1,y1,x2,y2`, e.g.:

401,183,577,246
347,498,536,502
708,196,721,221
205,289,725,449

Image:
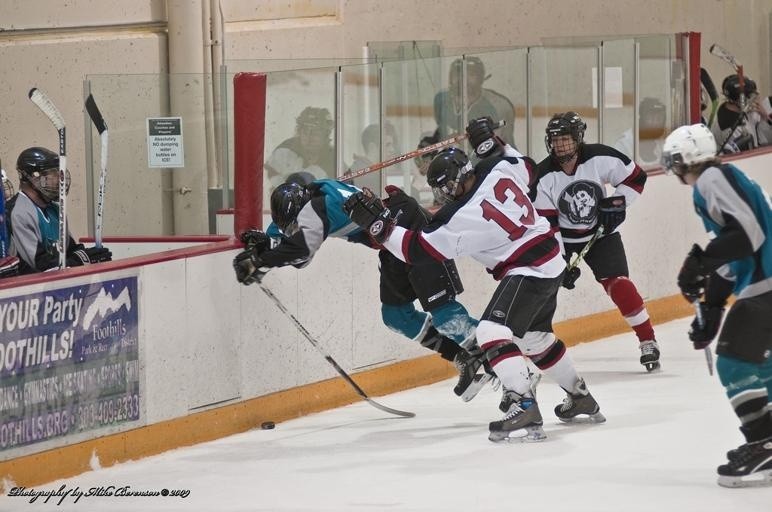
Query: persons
344,119,433,203
413,54,515,174
340,115,611,447
9,146,115,273
262,105,369,216
1,167,18,260
713,42,772,154
525,111,665,375
233,175,541,406
656,122,772,492
610,96,677,167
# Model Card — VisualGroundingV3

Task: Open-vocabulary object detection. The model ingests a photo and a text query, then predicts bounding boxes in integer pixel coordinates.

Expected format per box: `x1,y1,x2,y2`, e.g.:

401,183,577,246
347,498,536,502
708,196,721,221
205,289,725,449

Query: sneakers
454,348,600,431
718,443,772,477
639,339,660,364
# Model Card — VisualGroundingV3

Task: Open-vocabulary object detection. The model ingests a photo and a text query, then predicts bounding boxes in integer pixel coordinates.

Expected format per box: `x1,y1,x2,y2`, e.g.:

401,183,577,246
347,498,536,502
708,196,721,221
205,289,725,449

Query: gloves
343,187,398,246
466,117,498,158
597,196,625,234
72,246,112,265
688,272,734,349
679,244,710,303
233,246,272,286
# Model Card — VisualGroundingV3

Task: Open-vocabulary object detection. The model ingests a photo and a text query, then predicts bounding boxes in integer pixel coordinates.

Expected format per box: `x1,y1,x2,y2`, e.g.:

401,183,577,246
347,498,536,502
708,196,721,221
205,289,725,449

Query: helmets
270,182,310,225
660,124,716,176
639,98,666,129
426,147,472,205
449,57,485,114
295,107,333,151
723,75,759,103
544,112,586,164
16,147,71,202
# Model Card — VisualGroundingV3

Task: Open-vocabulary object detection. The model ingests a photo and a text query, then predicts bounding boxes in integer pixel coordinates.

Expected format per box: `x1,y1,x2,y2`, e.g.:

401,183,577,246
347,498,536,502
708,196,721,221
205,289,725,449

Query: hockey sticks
258,281,415,418
29,87,67,270
85,94,107,249
710,44,744,132
694,299,713,376
